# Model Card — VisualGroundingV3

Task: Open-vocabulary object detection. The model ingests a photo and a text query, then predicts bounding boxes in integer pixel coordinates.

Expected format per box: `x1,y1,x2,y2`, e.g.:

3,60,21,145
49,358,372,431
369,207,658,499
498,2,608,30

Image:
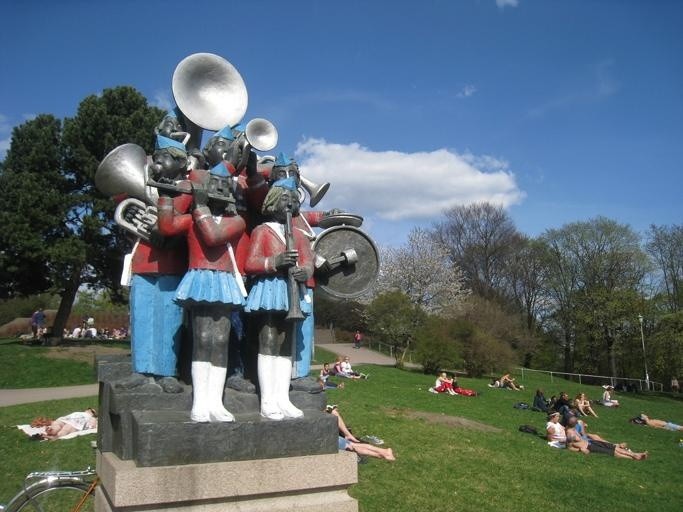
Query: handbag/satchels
628,416,645,425
513,401,530,409
518,424,537,435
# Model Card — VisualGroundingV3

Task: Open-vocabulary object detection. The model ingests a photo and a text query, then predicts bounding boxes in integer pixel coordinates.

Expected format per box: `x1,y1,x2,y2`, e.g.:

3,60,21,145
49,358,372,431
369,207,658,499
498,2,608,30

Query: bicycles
0,465,98,510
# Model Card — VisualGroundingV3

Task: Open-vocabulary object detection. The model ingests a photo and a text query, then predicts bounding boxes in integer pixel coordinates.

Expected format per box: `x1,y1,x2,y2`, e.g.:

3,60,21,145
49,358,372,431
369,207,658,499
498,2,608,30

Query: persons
433,372,474,396
43,407,97,441
319,356,368,390
534,387,649,461
635,412,681,431
490,371,521,389
324,405,397,461
32,309,128,341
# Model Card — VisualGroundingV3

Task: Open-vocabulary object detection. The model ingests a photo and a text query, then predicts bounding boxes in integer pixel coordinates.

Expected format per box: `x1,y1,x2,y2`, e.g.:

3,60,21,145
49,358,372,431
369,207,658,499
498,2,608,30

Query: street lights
636,313,650,389
